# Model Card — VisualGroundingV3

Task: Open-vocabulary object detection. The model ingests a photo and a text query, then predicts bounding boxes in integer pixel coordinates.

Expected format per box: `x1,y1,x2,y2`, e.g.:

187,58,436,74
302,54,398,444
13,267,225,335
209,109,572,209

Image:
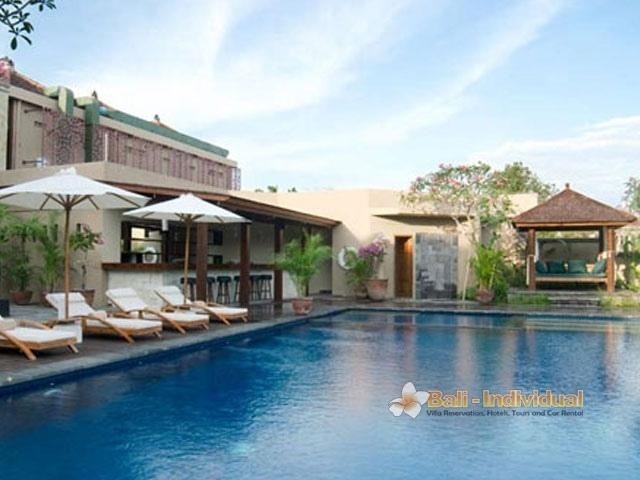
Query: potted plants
0,215,46,305
468,231,516,305
38,226,63,307
343,240,368,298
272,228,331,315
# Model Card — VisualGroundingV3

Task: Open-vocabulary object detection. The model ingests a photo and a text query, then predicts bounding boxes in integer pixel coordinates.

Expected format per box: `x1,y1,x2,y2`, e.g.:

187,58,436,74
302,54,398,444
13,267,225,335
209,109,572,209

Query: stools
180,276,272,302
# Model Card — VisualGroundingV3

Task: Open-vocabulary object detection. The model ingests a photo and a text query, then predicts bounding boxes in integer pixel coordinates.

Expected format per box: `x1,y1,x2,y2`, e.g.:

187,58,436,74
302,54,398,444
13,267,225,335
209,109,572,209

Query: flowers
359,236,389,279
69,223,104,288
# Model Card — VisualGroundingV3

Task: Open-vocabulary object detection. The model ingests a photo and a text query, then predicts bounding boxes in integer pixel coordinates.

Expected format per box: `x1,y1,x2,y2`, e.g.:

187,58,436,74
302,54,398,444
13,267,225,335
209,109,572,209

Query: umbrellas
123,193,253,305
0,168,153,319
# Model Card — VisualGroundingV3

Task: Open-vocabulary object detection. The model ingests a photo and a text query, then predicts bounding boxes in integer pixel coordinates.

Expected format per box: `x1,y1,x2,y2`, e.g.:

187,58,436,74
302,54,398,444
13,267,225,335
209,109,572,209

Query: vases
364,278,387,301
70,288,95,311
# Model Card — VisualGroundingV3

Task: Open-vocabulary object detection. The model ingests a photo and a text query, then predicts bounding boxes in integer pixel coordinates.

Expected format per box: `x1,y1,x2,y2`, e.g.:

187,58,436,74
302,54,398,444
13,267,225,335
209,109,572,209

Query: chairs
105,289,209,332
0,314,78,360
46,292,163,342
156,286,248,326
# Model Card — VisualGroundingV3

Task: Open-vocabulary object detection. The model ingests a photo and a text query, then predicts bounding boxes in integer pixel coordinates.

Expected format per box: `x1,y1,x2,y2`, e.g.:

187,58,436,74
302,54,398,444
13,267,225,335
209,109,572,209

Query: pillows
569,259,606,274
536,259,566,274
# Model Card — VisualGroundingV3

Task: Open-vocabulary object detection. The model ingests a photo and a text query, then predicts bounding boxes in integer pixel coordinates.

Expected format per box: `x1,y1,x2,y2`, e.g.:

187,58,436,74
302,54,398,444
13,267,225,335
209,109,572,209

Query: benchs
535,262,607,291
